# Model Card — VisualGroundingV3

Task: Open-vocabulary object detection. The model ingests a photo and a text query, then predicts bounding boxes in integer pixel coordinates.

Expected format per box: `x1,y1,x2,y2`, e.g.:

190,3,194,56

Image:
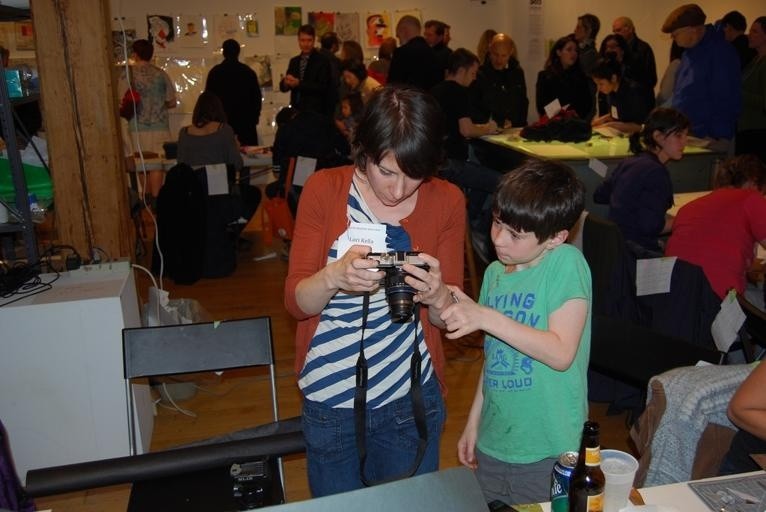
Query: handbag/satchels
120,87,141,121
519,108,592,141
240,197,294,245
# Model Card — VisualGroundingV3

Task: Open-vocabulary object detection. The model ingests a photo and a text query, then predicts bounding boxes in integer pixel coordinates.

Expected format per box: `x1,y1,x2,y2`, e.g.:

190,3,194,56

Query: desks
124,152,269,243
229,465,490,512
660,186,766,326
478,120,715,221
505,468,765,512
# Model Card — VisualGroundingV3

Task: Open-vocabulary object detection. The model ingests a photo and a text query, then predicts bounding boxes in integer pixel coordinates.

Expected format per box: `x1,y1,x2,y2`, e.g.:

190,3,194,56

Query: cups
598,448,640,511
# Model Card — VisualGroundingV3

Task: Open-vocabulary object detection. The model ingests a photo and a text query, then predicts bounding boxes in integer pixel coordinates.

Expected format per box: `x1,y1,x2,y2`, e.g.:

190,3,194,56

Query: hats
662,3,706,33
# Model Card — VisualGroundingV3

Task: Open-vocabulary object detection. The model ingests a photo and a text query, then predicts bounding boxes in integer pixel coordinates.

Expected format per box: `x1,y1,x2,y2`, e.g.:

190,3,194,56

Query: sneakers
471,230,488,264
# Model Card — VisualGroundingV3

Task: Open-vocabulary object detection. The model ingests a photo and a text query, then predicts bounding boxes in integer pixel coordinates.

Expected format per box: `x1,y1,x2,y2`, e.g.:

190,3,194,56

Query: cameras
366,251,430,323
230,461,272,508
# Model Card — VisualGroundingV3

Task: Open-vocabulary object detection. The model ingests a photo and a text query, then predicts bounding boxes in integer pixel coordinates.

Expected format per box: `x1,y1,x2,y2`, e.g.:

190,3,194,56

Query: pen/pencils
591,113,611,125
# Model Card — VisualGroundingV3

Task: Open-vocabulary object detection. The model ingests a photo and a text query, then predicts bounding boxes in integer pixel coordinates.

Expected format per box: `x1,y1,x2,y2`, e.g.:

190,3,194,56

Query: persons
177,91,262,235
270,3,766,219
719,355,766,474
441,159,592,506
120,39,176,208
284,85,466,500
595,107,690,257
204,39,263,182
666,153,766,344
427,49,504,265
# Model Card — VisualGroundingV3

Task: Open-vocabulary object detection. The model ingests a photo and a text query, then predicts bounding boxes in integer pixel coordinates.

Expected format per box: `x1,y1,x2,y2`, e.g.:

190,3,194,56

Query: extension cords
70,257,130,273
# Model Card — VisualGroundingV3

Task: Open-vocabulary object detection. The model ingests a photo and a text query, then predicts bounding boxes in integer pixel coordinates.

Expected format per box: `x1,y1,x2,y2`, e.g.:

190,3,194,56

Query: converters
67,254,80,270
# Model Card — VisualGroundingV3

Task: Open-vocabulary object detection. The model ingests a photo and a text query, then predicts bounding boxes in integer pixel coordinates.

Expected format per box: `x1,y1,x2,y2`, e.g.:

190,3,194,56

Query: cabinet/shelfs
0,257,154,488
0,61,53,272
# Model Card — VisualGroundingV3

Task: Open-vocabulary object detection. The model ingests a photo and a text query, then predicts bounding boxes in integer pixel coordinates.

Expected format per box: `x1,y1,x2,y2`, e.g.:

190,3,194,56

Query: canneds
550,451,580,512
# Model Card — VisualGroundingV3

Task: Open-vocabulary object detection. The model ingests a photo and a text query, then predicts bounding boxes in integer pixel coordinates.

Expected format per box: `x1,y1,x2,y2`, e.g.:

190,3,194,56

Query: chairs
619,238,755,370
578,211,634,418
119,313,288,512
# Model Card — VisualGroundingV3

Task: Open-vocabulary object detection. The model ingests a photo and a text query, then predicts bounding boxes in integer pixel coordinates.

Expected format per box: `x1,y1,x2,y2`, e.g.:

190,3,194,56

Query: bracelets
452,292,459,303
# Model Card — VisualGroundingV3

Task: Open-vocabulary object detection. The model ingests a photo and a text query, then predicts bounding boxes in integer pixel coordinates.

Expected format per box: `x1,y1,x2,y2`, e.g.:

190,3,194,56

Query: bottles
568,417,605,512
